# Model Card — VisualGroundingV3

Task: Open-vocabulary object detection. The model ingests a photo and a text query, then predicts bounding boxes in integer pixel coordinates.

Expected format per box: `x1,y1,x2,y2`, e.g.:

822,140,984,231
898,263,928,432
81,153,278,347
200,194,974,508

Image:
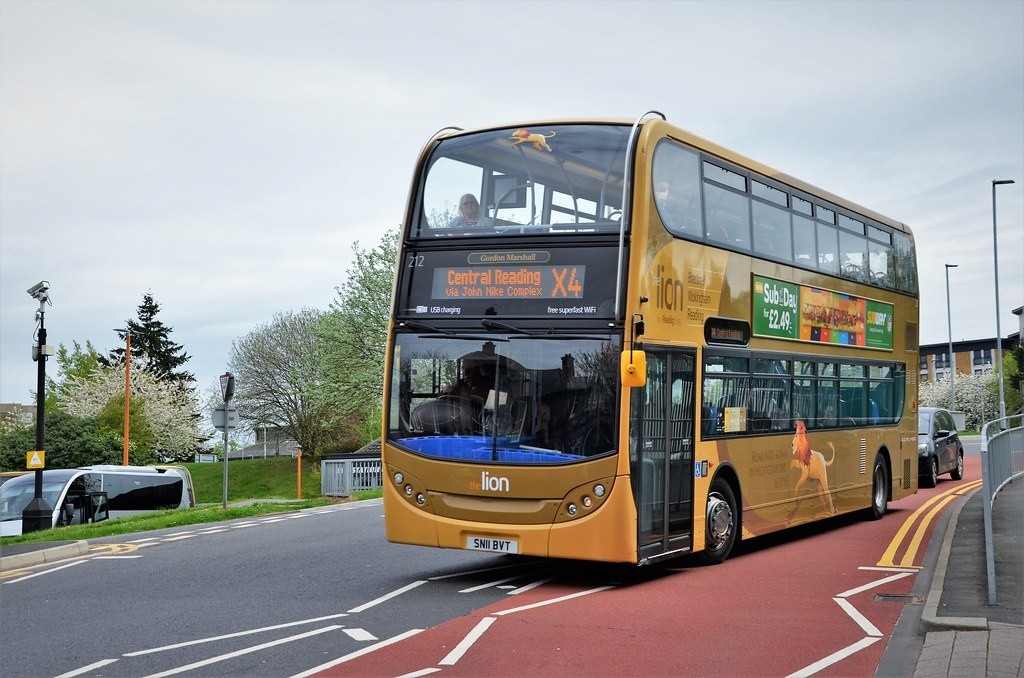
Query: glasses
460,201,477,206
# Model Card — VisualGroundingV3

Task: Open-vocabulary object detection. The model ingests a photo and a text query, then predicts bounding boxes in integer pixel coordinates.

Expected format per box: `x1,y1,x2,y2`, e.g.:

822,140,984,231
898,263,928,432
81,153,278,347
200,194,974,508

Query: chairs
701,387,880,434
798,255,887,285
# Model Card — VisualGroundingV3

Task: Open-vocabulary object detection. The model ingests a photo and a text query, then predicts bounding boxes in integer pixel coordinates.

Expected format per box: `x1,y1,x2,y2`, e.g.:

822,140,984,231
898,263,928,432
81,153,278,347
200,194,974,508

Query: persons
434,358,484,402
446,194,495,234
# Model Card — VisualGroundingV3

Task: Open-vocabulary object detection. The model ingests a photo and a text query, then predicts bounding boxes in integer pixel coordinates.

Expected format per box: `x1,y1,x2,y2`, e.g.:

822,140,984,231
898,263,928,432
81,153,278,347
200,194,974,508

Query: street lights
945,263,959,411
992,179,1015,431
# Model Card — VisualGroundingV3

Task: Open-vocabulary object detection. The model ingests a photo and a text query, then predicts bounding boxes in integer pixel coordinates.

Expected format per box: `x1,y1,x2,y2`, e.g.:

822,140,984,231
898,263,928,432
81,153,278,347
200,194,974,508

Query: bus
382,110,919,567
0,464,196,539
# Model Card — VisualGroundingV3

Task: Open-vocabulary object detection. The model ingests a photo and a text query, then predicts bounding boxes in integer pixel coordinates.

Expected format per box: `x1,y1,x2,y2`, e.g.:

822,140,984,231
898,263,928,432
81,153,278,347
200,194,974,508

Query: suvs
918,406,963,488
